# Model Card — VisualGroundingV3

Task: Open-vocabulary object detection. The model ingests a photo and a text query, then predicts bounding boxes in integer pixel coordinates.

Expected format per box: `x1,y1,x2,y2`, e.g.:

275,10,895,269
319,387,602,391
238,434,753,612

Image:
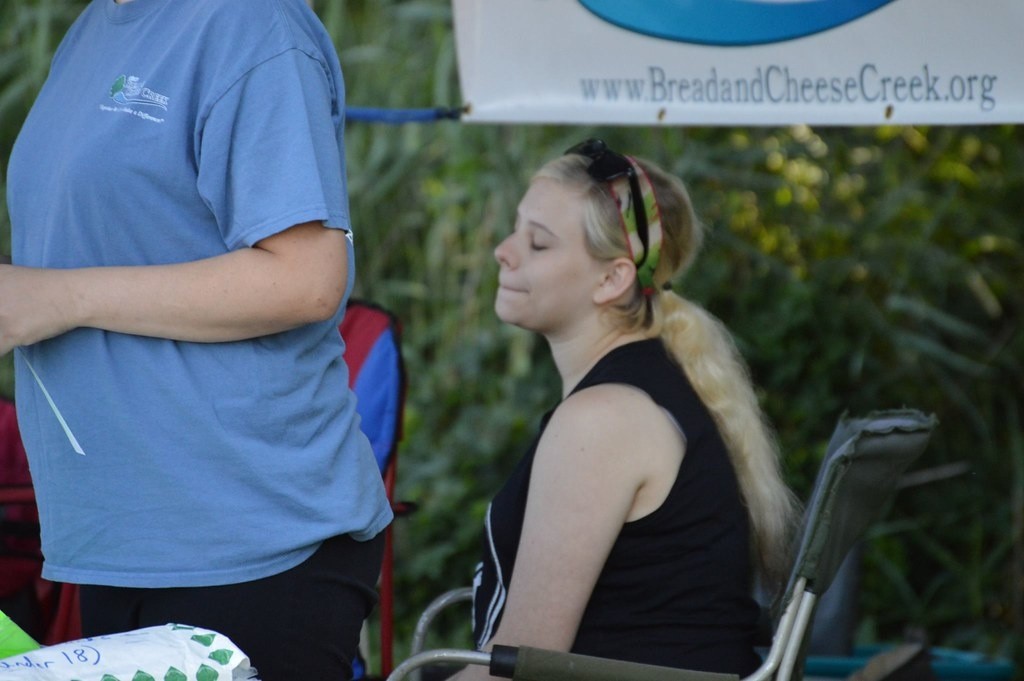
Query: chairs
389,412,935,681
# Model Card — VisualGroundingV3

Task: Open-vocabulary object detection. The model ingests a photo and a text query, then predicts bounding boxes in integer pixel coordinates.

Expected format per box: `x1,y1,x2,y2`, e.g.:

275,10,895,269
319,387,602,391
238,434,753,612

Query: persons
1,0,395,680
440,139,789,681
